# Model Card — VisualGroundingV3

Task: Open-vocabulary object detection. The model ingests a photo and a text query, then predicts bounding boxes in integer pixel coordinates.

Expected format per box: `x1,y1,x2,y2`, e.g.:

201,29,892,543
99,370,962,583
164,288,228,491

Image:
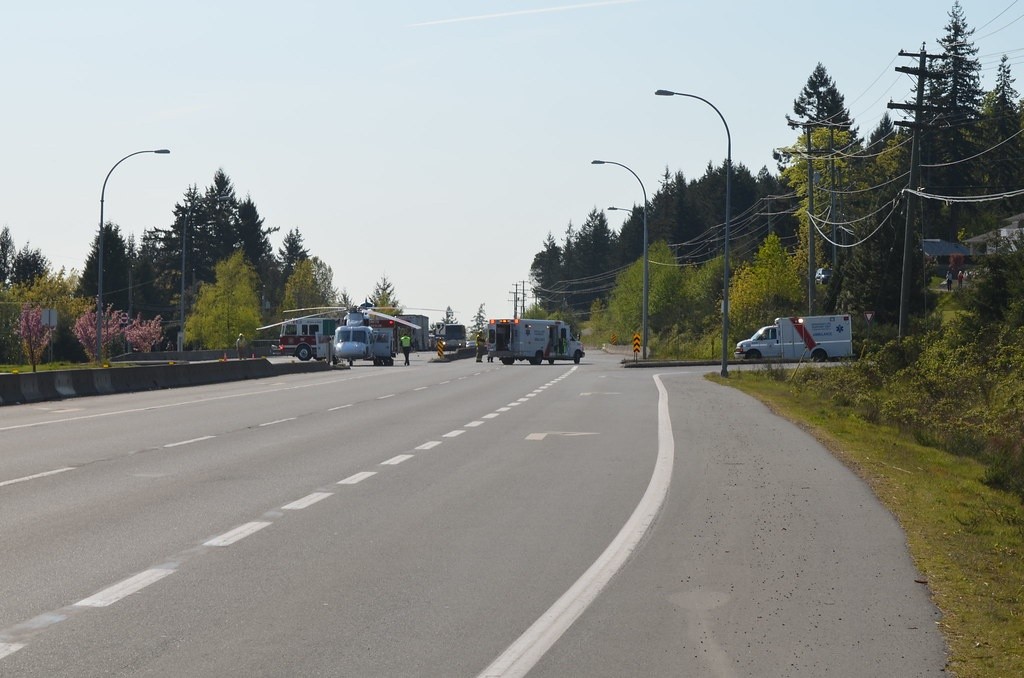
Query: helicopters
255,302,460,368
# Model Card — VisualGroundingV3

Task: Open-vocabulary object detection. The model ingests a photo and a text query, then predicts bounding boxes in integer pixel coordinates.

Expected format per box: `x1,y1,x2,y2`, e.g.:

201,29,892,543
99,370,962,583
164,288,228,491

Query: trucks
394,314,429,351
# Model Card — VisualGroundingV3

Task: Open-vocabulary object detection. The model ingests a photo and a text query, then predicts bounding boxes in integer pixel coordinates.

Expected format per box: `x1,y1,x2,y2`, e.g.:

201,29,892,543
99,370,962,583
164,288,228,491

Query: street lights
656,88,732,376
589,159,648,359
178,194,236,351
94,148,171,357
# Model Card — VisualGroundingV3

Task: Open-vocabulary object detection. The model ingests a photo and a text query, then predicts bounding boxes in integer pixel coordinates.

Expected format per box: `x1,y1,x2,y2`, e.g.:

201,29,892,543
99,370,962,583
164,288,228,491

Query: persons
476,331,494,363
235,333,248,360
399,333,414,366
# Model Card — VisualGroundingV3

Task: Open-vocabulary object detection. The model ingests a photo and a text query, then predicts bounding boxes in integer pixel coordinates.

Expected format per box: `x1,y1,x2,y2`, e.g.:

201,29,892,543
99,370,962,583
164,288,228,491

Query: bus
443,324,466,351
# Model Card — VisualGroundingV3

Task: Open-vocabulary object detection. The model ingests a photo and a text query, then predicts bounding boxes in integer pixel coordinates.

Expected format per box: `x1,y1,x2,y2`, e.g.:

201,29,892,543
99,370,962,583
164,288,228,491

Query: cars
429,335,435,350
815,267,834,285
466,341,476,347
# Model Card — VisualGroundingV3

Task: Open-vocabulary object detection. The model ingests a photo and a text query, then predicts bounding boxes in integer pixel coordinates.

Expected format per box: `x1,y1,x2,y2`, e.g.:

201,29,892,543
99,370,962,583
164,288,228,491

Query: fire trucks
275,317,397,365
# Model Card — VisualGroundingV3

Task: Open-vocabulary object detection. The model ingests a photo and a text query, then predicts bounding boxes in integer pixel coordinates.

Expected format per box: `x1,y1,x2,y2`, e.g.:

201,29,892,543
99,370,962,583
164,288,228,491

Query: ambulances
733,314,852,360
486,318,584,364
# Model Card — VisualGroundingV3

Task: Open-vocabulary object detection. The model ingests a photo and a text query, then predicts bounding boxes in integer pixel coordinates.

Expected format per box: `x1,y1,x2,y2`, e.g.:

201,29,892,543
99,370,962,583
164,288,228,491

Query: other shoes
476,359,494,363
404,362,410,366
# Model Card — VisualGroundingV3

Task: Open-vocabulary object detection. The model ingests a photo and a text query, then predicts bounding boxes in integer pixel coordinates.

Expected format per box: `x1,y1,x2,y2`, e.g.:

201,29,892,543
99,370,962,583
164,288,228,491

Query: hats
239,333,244,337
477,330,484,334
